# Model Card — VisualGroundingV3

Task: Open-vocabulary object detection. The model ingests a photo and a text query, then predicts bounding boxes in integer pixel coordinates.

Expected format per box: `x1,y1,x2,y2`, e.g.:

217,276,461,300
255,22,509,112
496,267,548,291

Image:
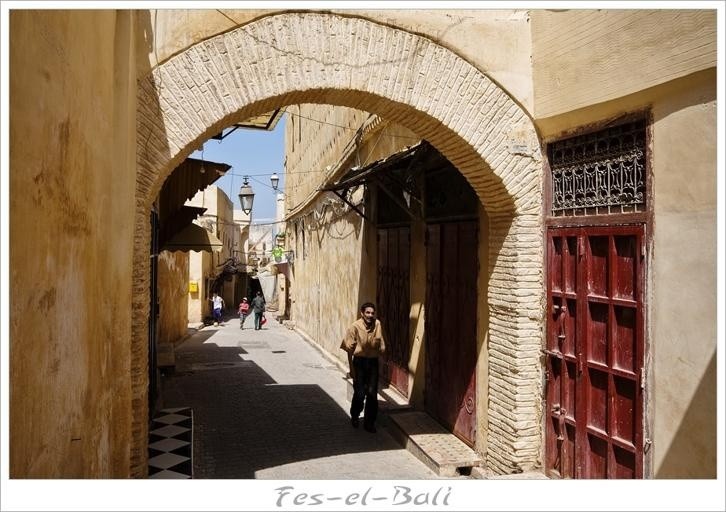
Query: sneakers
352,416,377,433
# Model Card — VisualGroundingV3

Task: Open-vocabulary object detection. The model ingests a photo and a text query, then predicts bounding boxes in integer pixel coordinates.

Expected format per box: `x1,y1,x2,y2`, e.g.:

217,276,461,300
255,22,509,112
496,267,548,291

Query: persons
237,297,249,330
250,291,265,330
341,302,387,433
208,291,225,323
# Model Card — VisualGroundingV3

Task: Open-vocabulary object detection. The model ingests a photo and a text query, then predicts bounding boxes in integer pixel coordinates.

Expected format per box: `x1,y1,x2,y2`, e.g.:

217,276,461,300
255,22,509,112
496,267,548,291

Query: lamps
239,176,255,215
200,151,206,173
270,174,279,191
